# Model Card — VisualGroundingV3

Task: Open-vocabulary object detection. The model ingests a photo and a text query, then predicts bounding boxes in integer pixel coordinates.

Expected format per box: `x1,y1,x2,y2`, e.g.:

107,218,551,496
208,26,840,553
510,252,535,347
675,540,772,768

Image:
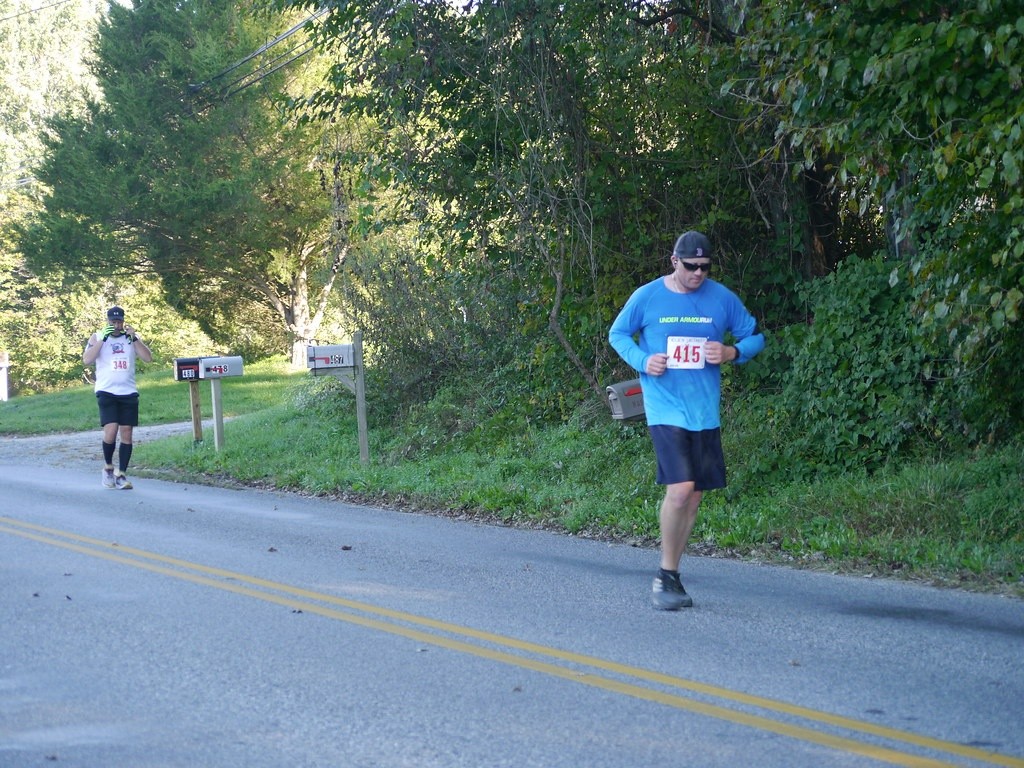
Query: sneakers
680,584,692,607
102,467,115,488
651,569,681,610
116,475,133,489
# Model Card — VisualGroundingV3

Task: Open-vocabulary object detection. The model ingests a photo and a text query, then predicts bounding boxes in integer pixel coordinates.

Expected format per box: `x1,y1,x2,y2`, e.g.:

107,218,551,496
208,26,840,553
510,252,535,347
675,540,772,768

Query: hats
674,231,711,259
107,307,125,321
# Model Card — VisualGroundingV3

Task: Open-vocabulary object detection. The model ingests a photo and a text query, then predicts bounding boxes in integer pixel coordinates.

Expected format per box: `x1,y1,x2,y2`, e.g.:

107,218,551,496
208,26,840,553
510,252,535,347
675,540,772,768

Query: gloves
101,324,116,342
120,330,132,344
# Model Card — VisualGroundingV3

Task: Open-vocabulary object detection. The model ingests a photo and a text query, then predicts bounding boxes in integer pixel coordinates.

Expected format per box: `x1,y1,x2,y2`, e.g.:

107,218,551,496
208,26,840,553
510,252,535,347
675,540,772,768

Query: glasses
679,258,712,272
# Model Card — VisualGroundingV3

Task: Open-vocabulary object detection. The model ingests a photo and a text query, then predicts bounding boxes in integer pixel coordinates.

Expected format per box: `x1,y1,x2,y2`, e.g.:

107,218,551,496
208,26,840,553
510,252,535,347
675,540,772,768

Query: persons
82,307,151,489
607,232,766,611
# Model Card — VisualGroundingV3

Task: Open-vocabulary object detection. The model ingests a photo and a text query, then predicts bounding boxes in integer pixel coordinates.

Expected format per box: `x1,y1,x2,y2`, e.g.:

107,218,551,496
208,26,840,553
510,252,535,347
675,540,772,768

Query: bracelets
132,336,138,342
